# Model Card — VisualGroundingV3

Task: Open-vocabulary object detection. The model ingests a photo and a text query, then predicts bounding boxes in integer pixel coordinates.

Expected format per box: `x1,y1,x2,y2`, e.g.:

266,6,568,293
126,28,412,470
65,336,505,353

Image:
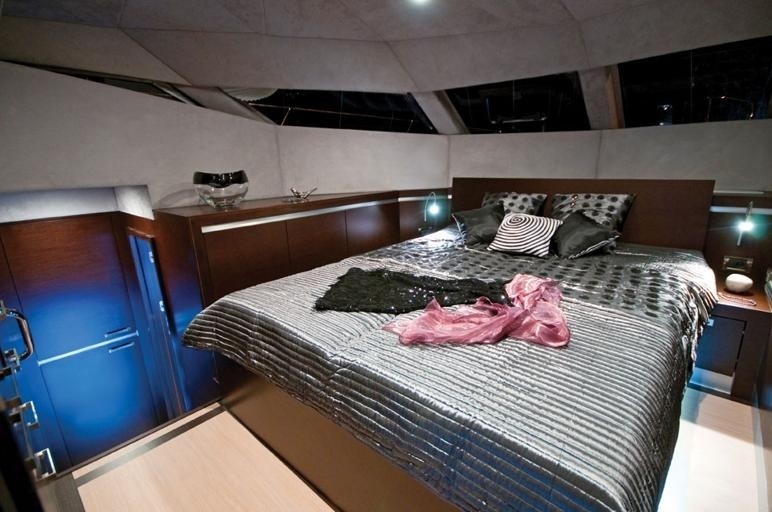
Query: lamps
735,201,754,247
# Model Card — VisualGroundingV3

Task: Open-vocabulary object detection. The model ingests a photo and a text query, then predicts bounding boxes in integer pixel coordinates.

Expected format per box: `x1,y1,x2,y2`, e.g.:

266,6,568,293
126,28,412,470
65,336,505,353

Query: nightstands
688,270,772,406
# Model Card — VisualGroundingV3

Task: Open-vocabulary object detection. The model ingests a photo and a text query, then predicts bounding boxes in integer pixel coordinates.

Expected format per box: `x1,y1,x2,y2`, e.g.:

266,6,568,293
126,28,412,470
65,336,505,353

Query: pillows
452,190,636,259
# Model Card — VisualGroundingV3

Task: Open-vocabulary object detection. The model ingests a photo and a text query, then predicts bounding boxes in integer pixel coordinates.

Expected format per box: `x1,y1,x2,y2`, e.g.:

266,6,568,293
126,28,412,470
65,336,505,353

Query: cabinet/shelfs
2,347,59,511
153,192,399,395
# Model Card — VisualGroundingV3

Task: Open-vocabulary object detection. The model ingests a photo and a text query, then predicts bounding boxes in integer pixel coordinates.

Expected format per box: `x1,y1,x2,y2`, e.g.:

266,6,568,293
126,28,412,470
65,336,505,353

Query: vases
193,170,249,209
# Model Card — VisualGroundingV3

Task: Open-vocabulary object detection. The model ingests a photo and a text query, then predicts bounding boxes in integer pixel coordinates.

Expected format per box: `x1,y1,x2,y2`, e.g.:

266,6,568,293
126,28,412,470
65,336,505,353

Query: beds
182,178,716,512
